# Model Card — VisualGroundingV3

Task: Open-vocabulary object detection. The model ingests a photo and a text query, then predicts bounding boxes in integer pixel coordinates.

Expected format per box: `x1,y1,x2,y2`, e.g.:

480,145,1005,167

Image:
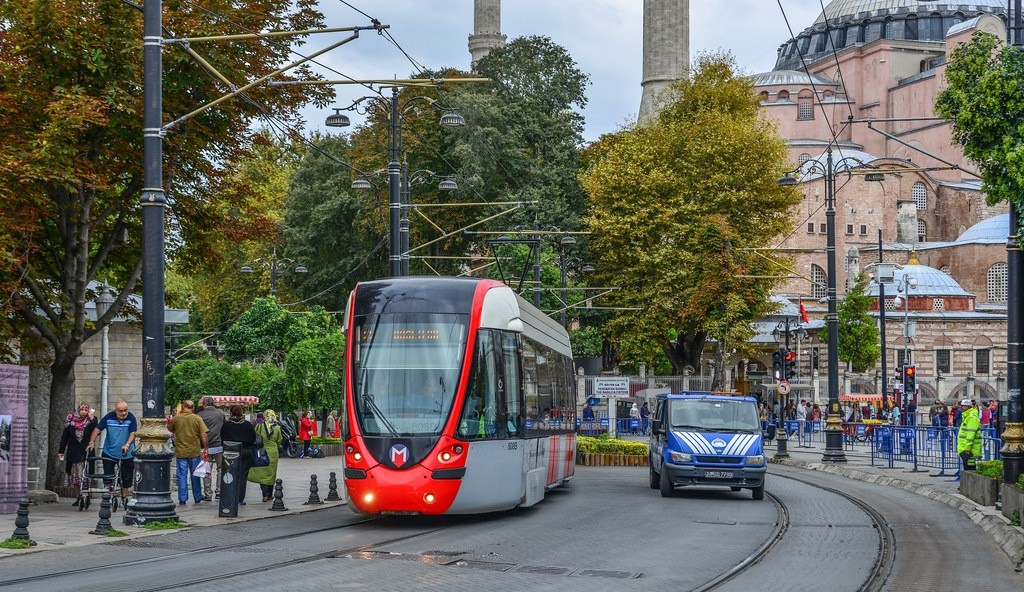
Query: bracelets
125,444,130,446
89,441,94,444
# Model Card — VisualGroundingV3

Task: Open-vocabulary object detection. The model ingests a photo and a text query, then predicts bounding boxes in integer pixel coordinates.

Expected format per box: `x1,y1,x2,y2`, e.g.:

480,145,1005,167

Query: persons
168,400,209,504
630,402,653,436
758,399,917,439
220,406,255,505
715,385,723,395
299,411,314,459
583,401,595,421
58,400,98,511
85,402,137,508
327,410,336,438
247,409,281,502
957,399,983,490
929,398,1000,455
414,373,450,415
164,397,226,501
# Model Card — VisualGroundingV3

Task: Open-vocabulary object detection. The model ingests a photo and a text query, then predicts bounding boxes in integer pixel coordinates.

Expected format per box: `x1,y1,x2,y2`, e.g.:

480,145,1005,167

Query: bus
336,237,576,514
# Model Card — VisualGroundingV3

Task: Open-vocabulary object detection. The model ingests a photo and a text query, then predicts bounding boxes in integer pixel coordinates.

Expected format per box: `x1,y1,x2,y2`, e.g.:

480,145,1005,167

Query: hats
961,399,972,406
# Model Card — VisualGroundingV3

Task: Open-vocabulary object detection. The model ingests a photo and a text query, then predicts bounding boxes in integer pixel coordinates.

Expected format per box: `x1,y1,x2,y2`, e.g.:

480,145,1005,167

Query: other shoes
204,495,211,501
263,496,274,502
303,456,312,458
179,500,186,505
195,495,203,503
240,501,246,505
215,494,220,499
108,496,114,505
119,501,124,507
73,498,79,506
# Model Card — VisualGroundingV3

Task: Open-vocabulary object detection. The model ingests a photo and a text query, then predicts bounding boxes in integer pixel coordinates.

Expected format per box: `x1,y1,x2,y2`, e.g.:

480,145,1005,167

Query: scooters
276,414,302,458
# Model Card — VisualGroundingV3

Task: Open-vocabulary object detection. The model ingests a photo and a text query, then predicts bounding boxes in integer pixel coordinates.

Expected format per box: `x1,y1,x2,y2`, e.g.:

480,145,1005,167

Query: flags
800,297,808,324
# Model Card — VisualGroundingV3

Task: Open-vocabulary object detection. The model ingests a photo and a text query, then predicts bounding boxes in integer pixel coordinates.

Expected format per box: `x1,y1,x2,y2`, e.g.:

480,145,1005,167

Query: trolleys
78,448,130,513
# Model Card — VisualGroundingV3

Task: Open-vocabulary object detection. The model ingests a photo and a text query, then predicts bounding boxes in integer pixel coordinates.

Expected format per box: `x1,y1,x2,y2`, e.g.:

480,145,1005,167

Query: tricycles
840,392,895,442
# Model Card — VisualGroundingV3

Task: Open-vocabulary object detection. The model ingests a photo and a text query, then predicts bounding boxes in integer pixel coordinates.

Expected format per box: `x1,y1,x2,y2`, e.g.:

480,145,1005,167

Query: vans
648,390,777,501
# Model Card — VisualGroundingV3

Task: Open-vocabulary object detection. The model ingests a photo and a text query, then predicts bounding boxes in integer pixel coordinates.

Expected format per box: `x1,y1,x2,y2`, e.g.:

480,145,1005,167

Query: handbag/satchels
251,438,270,466
253,433,264,448
193,460,213,479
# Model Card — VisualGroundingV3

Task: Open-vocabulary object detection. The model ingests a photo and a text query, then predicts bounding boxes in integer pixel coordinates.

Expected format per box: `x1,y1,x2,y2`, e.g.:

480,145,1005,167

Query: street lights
770,315,805,459
777,139,888,464
324,86,466,278
515,211,595,329
895,272,919,454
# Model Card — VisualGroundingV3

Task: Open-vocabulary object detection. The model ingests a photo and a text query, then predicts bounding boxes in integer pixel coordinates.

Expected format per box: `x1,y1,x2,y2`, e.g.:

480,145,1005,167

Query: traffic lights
784,348,796,379
896,365,904,383
772,351,783,380
904,365,916,394
